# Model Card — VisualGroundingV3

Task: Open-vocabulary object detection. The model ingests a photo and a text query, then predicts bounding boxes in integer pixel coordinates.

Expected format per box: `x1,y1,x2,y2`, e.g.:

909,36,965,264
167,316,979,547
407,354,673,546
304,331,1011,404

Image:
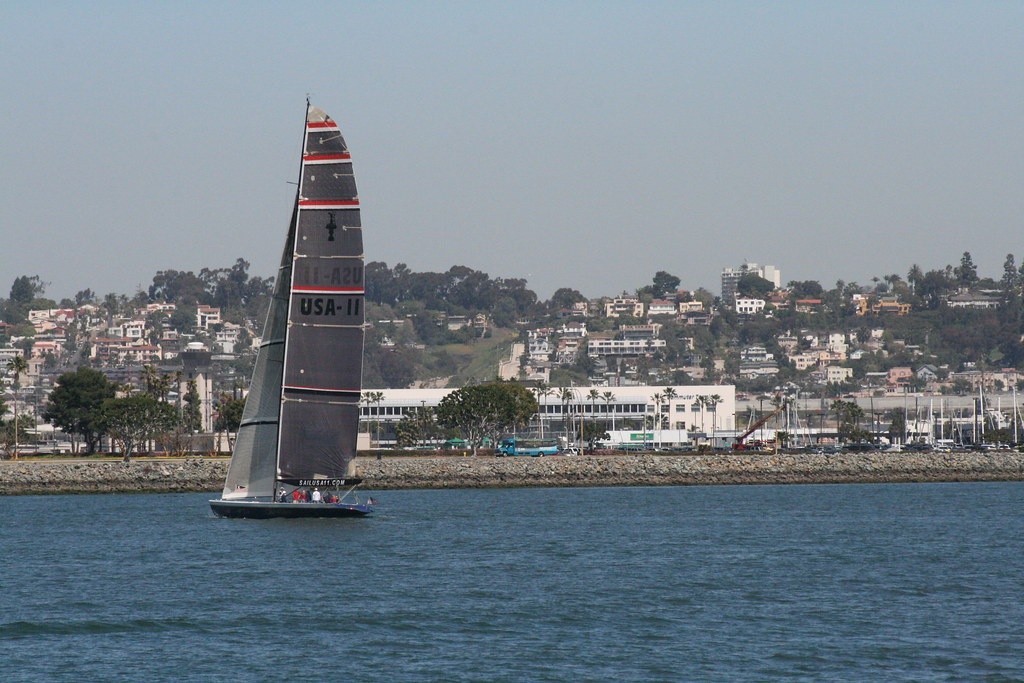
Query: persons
292,487,311,503
278,485,287,502
322,491,339,503
310,487,321,503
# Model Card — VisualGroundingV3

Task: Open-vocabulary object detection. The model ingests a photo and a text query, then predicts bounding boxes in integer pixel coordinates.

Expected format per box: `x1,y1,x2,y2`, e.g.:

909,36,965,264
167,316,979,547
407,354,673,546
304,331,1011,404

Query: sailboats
208,92,376,521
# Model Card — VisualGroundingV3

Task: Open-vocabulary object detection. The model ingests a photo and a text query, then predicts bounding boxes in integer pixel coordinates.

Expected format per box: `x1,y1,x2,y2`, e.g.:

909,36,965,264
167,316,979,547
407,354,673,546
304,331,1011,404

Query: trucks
495,438,560,458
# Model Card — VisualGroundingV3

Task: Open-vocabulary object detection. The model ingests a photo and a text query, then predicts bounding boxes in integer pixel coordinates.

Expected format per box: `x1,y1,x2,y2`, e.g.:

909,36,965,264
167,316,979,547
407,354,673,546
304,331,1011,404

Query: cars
805,440,1011,454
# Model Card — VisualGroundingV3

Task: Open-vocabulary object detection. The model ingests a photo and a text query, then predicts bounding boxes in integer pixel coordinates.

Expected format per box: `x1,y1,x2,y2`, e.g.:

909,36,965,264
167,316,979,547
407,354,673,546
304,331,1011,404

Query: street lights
873,411,883,449
817,413,827,446
421,400,427,451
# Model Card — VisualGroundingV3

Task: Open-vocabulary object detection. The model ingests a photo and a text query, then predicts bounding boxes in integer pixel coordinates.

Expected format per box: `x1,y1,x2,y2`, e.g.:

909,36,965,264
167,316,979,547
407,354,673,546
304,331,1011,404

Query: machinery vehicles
731,405,786,452
